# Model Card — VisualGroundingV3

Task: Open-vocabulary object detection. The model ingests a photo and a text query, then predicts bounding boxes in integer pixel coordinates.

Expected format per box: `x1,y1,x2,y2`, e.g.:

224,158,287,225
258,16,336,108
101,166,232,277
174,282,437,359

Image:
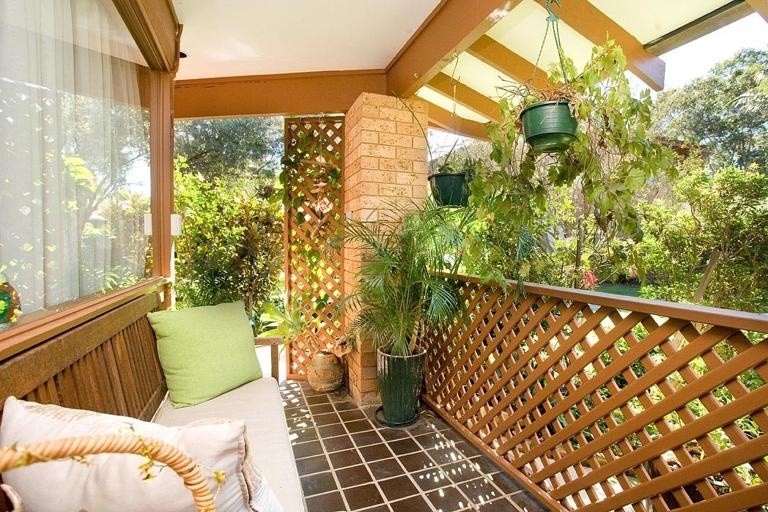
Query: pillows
1,393,251,511
147,300,260,409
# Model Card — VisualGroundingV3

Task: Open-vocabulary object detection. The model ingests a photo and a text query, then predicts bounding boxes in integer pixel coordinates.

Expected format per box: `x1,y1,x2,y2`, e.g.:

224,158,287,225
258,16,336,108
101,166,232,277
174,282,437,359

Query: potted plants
413,150,538,276
490,35,673,243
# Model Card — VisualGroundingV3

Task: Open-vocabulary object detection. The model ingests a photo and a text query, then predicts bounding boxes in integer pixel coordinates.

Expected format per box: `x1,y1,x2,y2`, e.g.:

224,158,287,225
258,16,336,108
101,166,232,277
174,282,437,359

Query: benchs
0,275,312,512
